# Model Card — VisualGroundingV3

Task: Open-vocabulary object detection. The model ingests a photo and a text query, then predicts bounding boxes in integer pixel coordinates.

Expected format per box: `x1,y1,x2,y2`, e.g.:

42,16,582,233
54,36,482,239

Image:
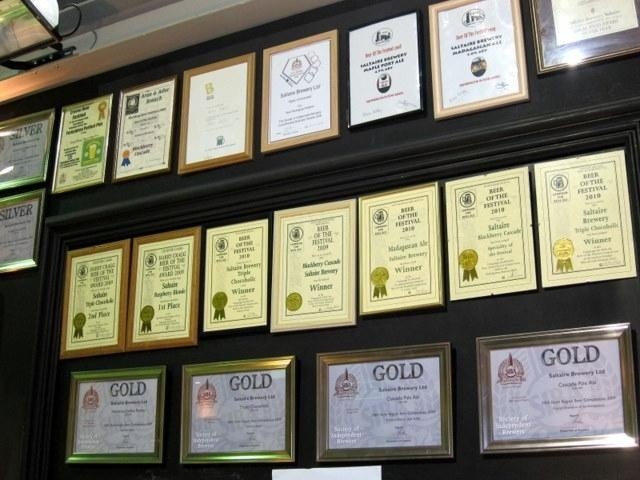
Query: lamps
0,0,82,71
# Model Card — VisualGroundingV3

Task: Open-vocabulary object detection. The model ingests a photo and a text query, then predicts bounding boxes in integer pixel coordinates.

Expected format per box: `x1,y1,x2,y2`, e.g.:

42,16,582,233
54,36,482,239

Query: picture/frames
203,198,357,332
60,225,202,358
0,194,46,276
46,90,114,192
356,180,444,316
476,323,639,454
422,1,532,121
1,110,58,188
315,342,454,463
445,148,637,302
526,0,640,84
118,69,177,181
179,355,294,464
253,21,338,155
175,48,256,174
346,8,428,132
63,365,164,463
